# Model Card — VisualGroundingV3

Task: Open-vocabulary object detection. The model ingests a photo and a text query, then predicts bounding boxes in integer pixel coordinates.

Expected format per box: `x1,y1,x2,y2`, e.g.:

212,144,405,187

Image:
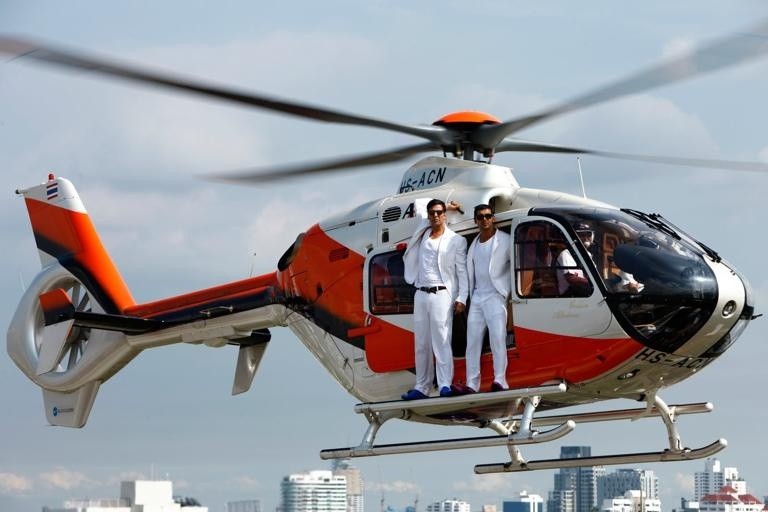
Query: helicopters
0,16,768,476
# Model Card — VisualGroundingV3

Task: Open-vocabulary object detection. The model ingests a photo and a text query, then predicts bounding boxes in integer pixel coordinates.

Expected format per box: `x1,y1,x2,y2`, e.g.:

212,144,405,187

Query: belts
417,286,447,294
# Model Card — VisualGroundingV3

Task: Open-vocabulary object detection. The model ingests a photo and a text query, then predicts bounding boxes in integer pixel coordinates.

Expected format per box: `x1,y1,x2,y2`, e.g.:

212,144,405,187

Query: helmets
574,223,594,248
639,233,660,249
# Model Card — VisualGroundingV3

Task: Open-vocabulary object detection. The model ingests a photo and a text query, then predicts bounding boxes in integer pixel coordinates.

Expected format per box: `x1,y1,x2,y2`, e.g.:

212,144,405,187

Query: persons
617,270,644,294
399,200,470,400
555,219,594,296
448,203,517,397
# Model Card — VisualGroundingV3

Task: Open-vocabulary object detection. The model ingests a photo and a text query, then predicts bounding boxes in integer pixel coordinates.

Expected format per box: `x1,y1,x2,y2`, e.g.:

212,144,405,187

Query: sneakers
491,382,504,391
450,385,475,395
401,390,428,399
440,387,452,396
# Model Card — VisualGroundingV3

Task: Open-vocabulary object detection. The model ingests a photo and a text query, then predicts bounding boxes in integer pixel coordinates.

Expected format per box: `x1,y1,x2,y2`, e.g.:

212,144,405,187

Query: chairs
533,242,558,296
370,263,413,313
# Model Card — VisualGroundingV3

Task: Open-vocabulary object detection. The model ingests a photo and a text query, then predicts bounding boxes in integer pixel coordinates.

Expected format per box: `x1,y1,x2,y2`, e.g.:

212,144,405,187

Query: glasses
429,210,442,215
476,214,494,219
